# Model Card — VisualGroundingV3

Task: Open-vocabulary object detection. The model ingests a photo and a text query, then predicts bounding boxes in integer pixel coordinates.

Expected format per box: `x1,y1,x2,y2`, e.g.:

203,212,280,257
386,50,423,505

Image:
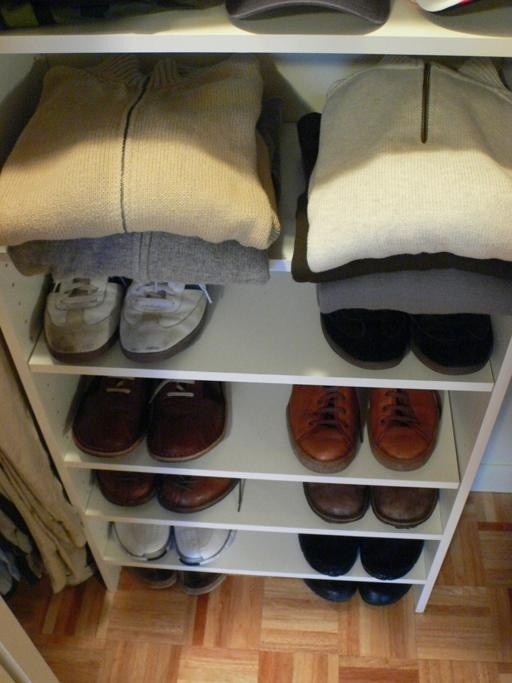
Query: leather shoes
173,524,234,564
288,384,363,474
112,523,171,559
371,485,439,527
96,467,154,507
368,388,441,472
156,476,239,514
302,481,370,524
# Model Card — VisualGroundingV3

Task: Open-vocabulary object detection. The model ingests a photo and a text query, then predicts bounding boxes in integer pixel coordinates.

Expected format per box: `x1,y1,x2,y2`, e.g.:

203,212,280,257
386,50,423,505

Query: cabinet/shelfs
0,0,512,614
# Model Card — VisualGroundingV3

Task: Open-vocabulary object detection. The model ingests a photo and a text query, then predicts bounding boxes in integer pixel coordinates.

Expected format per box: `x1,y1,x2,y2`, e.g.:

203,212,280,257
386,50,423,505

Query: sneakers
119,279,214,364
44,276,125,363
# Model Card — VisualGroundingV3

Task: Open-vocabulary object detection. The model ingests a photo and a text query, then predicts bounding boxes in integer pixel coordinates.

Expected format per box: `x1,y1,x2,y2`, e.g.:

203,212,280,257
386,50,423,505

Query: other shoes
147,373,227,463
405,310,493,374
320,311,410,370
358,580,413,603
304,578,359,602
360,536,427,582
176,572,224,596
297,534,360,577
131,565,175,589
73,373,147,459
